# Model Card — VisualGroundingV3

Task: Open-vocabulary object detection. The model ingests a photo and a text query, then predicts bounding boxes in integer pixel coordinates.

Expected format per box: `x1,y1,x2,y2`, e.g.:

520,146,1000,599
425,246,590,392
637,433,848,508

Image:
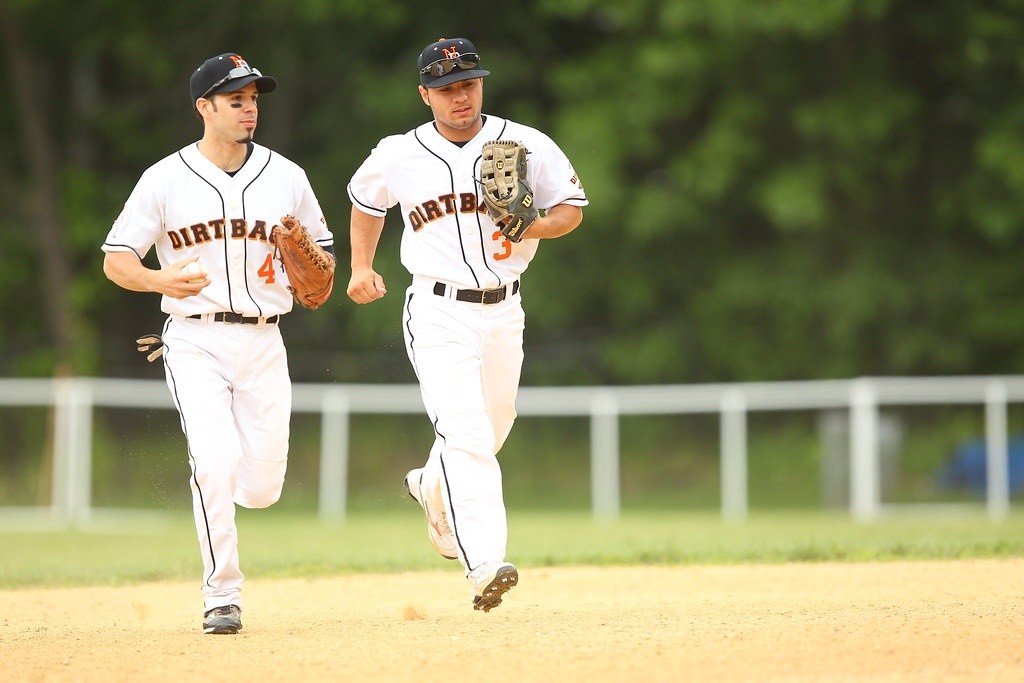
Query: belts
432,280,519,304
188,312,280,325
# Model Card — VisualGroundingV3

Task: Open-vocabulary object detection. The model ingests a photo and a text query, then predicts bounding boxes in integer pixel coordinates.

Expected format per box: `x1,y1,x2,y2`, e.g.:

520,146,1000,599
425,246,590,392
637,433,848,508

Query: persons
346,36,589,614
102,53,338,633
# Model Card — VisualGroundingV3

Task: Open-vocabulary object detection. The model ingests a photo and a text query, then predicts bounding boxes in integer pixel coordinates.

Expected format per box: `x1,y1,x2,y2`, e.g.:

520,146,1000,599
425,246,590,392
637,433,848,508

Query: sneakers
471,560,518,611
404,466,460,560
202,604,243,636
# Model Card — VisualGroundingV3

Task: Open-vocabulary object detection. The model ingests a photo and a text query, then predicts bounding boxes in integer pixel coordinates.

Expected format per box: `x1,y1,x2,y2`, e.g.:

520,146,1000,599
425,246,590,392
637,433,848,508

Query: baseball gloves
479,139,539,243
270,215,338,311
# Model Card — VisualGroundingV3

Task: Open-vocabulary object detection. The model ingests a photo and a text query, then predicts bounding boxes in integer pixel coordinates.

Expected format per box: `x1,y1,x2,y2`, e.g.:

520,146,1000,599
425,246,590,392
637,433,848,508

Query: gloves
136,334,163,362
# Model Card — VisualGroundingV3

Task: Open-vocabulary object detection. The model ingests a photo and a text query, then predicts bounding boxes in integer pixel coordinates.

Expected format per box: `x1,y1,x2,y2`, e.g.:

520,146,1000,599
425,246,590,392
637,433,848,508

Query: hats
418,38,490,89
190,52,276,112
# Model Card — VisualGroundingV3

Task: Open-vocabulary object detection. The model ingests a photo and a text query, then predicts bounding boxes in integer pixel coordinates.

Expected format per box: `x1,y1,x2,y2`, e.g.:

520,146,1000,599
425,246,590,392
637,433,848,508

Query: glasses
420,53,481,77
201,66,262,96
